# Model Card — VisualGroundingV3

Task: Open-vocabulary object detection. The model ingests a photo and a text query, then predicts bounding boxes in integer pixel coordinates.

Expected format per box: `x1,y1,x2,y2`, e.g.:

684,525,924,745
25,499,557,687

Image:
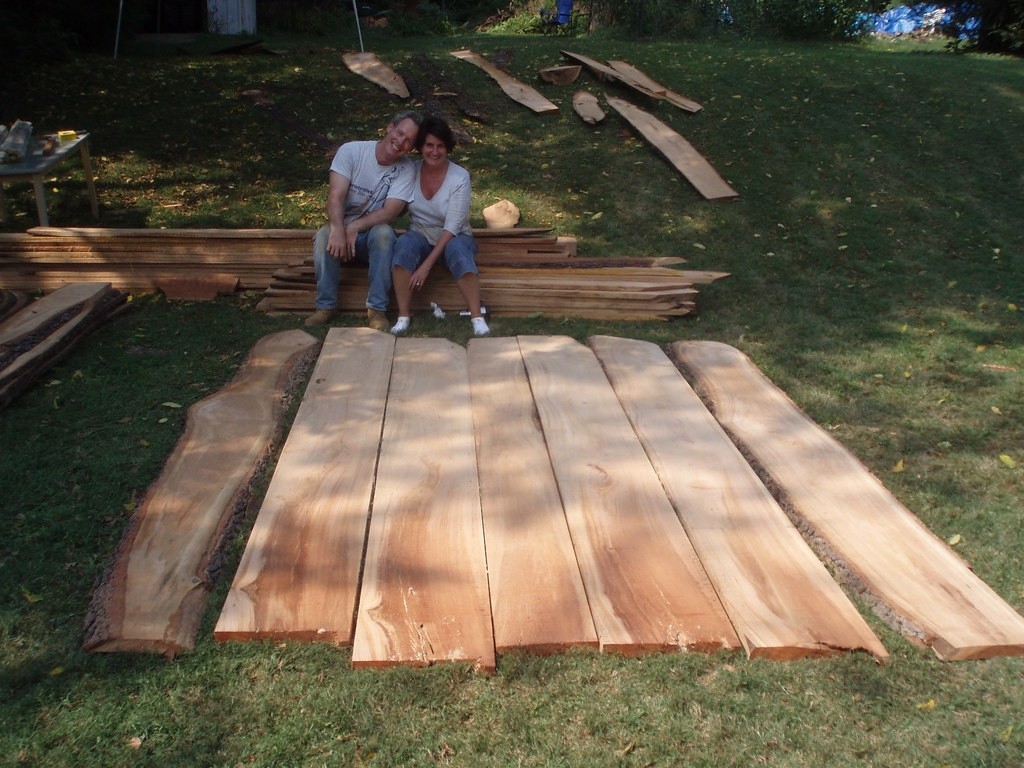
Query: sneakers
368,308,391,333
471,317,490,336
305,309,336,326
391,317,411,334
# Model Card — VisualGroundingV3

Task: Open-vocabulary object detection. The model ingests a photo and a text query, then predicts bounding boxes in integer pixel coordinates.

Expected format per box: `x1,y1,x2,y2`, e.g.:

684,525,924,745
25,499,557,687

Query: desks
0,133,99,227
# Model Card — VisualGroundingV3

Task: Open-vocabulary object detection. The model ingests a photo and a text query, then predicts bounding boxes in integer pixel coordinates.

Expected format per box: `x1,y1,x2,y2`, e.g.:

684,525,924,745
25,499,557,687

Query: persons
389,118,483,336
304,111,422,330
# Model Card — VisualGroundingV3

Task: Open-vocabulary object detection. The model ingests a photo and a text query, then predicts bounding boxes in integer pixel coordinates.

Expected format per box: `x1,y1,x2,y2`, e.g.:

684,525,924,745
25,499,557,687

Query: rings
416,283,420,285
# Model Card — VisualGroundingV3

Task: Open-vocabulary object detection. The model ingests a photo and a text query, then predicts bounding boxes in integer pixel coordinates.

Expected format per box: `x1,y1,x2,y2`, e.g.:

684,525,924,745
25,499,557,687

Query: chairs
539,0,574,38
